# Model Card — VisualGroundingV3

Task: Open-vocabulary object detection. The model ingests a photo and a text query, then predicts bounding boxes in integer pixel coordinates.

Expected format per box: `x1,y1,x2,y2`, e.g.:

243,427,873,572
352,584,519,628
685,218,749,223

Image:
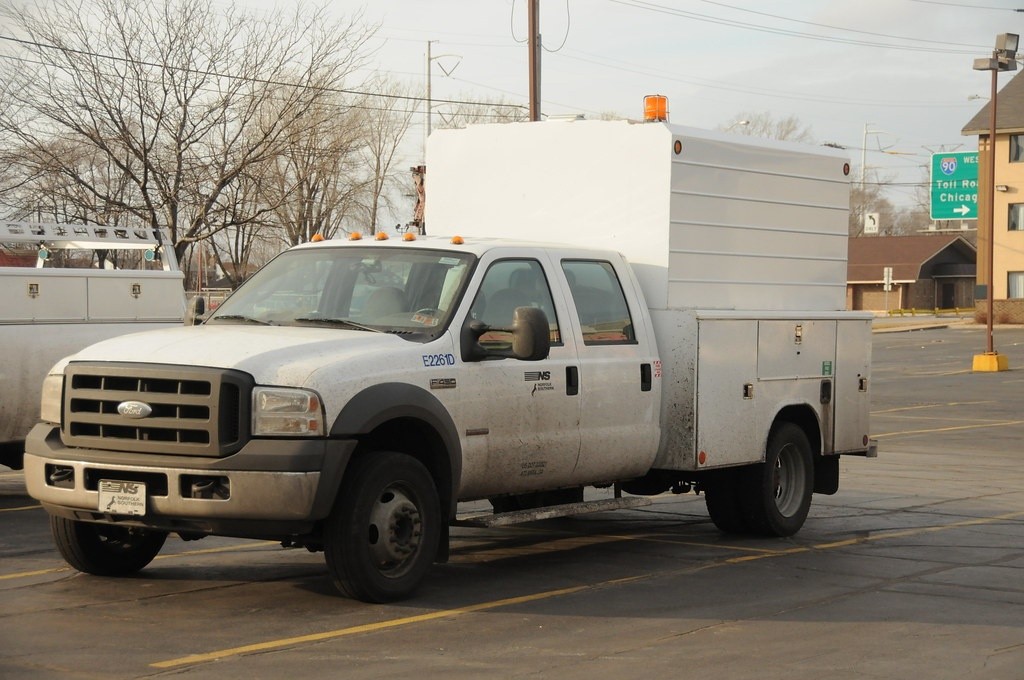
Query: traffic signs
929,153,979,221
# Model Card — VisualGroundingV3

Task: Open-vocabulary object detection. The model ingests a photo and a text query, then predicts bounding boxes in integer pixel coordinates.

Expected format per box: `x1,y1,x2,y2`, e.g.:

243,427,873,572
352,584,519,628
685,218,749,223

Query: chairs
482,290,528,331
365,289,406,320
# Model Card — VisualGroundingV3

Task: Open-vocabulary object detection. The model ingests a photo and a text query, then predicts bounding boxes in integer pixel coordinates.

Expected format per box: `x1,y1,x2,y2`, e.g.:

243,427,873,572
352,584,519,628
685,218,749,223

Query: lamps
996,185,1009,192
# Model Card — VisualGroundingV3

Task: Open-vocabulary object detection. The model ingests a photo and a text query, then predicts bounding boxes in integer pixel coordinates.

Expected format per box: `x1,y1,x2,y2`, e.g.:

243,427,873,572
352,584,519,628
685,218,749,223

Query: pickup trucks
21,92,879,603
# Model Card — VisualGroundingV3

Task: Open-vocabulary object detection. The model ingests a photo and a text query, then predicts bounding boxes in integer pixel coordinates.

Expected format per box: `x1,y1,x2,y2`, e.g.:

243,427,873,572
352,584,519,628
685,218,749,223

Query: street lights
971,32,1020,372
